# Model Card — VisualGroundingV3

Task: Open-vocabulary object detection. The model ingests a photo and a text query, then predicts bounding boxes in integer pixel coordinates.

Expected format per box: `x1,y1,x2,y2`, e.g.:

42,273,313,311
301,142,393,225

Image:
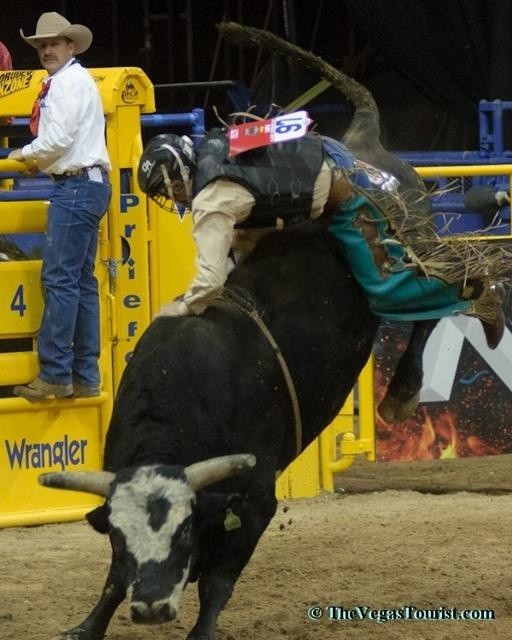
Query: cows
37,20,441,640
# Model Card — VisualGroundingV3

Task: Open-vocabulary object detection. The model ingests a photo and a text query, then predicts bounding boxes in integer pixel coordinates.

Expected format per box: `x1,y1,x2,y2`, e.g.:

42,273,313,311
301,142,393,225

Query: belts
51,165,105,180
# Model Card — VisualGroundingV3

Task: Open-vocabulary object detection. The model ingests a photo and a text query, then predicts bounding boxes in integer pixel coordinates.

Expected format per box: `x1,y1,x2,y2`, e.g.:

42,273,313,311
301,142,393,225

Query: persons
137,108,511,351
464,166,512,227
5,10,116,401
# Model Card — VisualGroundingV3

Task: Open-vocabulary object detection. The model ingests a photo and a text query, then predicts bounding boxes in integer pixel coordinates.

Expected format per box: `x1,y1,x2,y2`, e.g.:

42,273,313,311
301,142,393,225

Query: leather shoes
477,279,506,350
14,374,99,398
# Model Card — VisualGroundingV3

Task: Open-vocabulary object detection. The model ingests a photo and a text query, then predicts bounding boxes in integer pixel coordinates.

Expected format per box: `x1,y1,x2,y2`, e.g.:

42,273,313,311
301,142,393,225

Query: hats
20,12,94,55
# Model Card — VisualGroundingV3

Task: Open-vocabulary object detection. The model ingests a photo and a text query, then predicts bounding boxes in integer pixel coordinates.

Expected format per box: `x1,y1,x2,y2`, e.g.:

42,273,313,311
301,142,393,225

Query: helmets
138,133,195,198
